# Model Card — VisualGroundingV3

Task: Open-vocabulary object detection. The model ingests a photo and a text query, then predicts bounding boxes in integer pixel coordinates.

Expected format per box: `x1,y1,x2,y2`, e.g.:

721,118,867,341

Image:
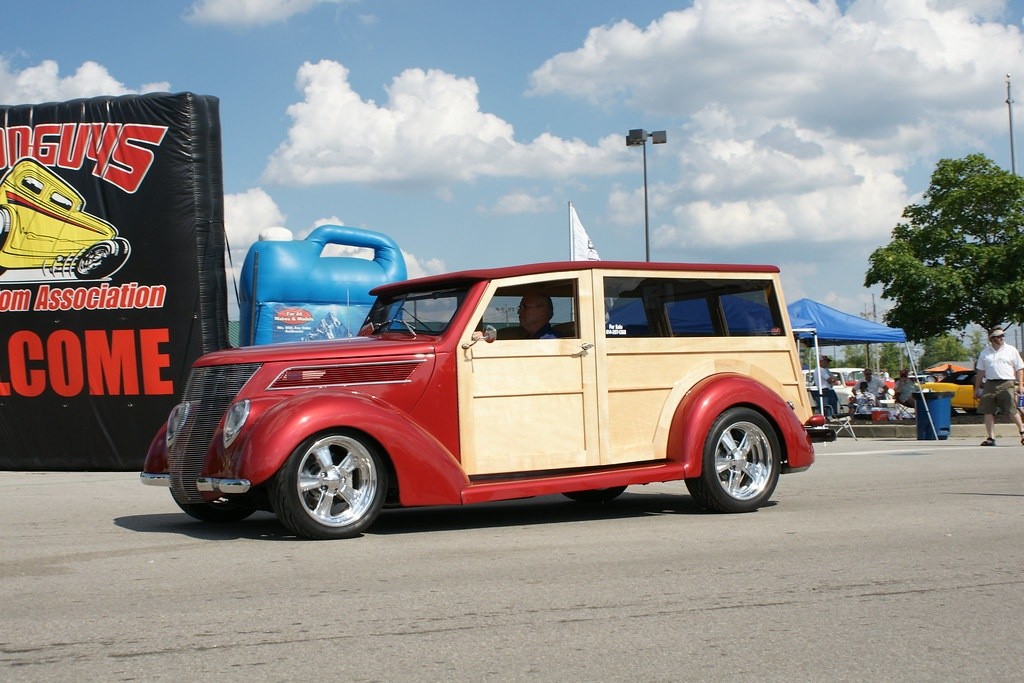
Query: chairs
550,320,575,337
822,405,858,441
495,326,529,340
892,386,914,419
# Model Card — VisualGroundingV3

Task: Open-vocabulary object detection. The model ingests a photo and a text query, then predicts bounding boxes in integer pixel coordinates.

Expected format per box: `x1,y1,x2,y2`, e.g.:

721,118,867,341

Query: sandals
1020,431,1024,445
981,438,996,446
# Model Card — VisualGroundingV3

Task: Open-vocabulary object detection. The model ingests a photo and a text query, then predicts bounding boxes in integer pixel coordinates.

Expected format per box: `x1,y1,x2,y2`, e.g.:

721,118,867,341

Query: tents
786,297,939,441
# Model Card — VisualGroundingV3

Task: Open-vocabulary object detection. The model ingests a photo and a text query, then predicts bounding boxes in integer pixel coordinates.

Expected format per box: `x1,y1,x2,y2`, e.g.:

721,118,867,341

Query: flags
572,206,602,261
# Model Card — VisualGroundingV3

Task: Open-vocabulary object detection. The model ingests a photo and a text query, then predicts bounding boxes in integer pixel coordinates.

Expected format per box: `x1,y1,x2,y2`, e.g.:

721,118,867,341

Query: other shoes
866,420,872,425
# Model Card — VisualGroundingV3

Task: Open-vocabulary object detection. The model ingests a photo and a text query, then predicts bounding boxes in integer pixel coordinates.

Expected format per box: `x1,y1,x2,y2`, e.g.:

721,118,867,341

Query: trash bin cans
913,391,954,440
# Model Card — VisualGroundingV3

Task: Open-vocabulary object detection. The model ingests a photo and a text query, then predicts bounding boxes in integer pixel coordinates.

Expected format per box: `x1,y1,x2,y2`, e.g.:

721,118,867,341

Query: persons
518,287,560,339
849,368,889,421
945,364,954,376
893,369,919,408
973,328,1024,446
810,355,838,416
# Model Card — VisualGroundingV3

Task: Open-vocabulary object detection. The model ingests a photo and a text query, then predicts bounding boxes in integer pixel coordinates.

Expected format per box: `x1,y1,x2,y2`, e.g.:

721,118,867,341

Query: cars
138,261,841,542
800,366,1024,417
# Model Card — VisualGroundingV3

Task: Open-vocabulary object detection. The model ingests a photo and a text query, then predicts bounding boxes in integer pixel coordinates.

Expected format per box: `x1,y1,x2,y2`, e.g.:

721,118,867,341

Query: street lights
625,129,668,261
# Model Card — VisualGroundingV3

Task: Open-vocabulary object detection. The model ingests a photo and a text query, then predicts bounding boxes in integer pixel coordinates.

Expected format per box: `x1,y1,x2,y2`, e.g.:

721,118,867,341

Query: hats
900,369,908,374
948,363,952,365
990,330,1006,337
821,356,832,361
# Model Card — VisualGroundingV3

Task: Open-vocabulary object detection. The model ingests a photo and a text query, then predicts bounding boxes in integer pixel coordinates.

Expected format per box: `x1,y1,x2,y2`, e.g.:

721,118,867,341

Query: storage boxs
871,406,889,422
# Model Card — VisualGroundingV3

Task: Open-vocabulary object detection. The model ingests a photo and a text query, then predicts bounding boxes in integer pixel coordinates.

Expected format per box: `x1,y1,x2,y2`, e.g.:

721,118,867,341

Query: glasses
519,304,547,309
901,375,907,378
